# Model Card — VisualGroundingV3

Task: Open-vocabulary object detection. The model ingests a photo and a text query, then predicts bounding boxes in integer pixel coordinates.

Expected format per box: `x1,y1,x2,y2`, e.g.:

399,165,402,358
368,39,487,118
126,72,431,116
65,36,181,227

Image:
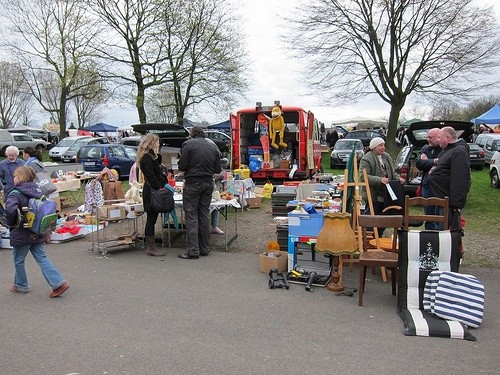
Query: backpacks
10,189,57,235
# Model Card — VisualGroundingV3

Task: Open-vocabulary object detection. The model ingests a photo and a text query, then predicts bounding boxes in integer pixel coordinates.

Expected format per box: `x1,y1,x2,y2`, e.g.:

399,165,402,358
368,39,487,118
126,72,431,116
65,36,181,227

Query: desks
162,193,240,252
50,175,95,208
224,177,255,215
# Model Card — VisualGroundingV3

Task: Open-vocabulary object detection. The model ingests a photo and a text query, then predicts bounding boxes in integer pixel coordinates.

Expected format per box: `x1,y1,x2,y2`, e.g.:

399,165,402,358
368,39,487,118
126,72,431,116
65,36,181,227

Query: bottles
21,206,29,228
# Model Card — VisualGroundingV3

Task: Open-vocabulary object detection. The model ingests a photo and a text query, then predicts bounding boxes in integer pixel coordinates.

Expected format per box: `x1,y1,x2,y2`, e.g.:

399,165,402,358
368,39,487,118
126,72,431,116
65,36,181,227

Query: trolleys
88,201,143,256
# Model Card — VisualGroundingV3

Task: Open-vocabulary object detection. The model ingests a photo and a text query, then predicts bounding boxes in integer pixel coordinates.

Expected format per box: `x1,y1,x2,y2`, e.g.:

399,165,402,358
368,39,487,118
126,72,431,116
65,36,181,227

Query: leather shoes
179,252,209,258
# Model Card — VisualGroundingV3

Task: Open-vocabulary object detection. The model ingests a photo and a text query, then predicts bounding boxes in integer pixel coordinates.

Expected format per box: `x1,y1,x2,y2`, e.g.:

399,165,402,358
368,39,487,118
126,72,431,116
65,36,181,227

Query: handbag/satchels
150,187,175,213
30,163,57,195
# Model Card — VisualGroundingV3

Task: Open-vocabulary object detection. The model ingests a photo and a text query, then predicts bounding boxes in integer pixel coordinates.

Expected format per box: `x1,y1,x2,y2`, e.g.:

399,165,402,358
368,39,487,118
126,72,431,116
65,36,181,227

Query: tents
208,120,231,132
6,124,48,142
84,123,119,145
175,118,197,127
469,103,500,132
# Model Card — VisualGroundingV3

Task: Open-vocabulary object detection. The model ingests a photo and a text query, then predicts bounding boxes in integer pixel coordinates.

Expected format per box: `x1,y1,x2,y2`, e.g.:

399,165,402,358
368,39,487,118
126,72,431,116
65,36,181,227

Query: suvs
81,145,144,181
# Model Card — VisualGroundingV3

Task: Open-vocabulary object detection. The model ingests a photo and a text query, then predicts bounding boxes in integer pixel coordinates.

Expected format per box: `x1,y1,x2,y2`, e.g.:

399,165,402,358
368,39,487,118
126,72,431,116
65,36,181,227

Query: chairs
398,229,477,340
404,195,449,232
357,215,404,306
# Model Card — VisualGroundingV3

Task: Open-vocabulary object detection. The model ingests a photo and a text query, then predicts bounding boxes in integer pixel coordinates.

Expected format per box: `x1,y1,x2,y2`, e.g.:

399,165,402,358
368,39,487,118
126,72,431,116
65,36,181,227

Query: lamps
314,211,359,292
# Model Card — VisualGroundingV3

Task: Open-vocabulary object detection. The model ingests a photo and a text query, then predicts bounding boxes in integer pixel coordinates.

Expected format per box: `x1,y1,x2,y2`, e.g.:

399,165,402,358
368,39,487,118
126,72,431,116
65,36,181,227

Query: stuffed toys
269,105,287,148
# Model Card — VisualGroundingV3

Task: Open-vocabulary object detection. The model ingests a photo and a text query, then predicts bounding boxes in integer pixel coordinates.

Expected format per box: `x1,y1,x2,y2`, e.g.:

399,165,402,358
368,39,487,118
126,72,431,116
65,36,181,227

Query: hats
368,137,386,149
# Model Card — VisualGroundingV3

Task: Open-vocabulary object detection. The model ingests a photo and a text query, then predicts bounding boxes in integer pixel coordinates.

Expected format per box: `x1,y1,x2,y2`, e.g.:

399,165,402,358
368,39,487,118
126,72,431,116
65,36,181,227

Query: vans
61,138,110,165
201,131,232,154
49,136,91,162
229,101,325,185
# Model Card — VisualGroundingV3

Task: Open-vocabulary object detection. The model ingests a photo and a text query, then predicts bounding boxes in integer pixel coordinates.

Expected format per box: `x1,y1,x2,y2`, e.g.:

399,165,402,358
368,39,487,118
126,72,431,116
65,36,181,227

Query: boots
145,235,166,256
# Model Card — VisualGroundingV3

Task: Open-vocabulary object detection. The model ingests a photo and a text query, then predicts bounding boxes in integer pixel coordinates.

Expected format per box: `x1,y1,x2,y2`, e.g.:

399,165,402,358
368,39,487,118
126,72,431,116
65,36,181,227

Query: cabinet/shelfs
91,199,145,255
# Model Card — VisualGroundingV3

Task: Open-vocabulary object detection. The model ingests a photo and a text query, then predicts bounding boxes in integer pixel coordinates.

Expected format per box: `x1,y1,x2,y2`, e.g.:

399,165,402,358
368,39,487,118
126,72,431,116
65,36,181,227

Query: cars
465,141,485,170
395,120,475,198
344,130,402,151
122,136,143,147
476,132,500,163
489,148,500,187
133,123,194,189
330,139,366,169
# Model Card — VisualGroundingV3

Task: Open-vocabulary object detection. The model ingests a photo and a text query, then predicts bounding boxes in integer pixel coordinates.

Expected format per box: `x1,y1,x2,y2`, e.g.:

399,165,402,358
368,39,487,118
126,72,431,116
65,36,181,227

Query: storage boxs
288,210,325,238
97,204,126,222
257,249,288,275
234,165,251,180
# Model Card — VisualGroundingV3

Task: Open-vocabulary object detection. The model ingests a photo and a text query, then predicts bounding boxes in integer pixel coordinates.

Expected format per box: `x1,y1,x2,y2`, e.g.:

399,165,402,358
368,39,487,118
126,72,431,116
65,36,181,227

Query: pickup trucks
0,133,47,157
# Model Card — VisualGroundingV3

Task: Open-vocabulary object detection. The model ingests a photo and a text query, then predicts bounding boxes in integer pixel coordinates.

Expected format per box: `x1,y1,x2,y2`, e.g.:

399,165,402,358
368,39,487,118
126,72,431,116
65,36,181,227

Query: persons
177,127,221,259
0,146,25,203
3,167,69,299
22,146,45,173
428,126,473,230
137,133,169,257
212,167,226,234
415,127,445,231
358,136,406,240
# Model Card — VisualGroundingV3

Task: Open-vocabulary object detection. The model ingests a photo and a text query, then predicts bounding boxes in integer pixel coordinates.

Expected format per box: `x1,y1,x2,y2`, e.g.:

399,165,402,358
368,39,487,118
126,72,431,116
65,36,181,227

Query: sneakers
211,226,225,234
49,283,70,298
8,285,29,293
